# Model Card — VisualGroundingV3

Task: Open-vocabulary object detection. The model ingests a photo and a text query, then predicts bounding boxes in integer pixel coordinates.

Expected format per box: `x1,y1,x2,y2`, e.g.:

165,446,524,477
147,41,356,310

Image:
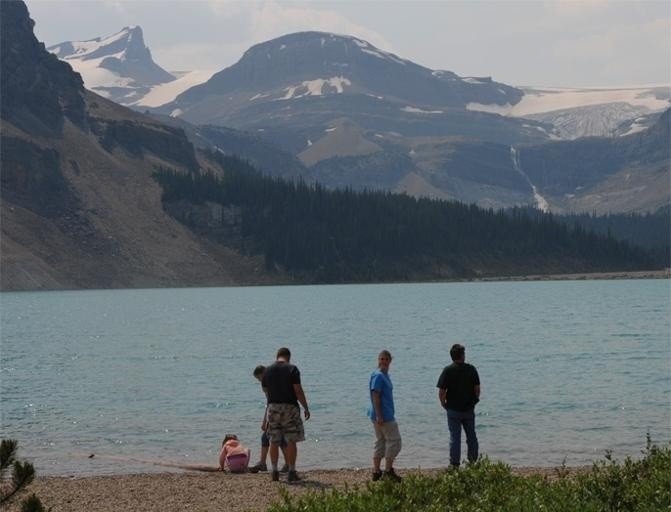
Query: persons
365,348,405,485
260,346,313,483
254,363,291,473
220,432,255,474
438,342,483,475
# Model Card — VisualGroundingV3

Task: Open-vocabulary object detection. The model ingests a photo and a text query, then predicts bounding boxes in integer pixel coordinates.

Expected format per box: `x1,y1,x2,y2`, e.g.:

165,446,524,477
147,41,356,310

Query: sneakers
249,463,268,473
272,470,280,481
373,466,405,483
288,469,302,482
280,463,290,472
